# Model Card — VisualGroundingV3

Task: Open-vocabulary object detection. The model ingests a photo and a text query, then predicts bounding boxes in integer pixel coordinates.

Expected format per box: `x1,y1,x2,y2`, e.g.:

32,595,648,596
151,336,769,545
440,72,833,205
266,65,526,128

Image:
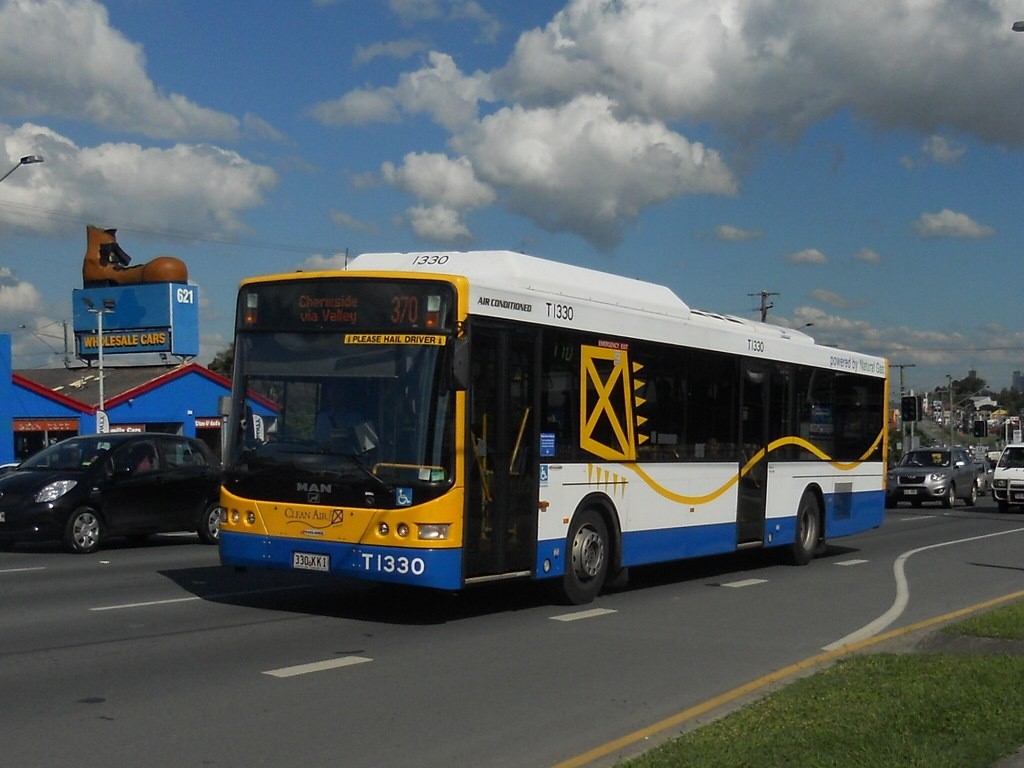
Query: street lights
84,298,116,451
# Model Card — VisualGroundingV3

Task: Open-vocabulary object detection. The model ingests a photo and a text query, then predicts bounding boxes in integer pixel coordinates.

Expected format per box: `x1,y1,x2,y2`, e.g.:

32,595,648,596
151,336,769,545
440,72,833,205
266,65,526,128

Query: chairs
191,452,204,465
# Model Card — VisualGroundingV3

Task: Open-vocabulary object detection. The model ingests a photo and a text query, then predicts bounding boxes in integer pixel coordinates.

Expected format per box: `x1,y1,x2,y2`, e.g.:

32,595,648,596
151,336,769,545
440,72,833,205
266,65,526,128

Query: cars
971,460,995,495
808,411,834,440
938,415,952,426
0,432,221,553
989,442,1024,513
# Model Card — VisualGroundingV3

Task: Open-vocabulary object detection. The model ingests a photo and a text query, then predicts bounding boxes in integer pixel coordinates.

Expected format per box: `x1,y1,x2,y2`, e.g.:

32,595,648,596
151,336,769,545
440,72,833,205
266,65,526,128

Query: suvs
885,446,978,509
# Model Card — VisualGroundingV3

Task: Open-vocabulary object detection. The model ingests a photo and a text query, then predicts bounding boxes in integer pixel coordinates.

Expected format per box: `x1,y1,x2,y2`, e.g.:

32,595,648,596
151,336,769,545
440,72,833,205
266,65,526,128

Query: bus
219,251,888,604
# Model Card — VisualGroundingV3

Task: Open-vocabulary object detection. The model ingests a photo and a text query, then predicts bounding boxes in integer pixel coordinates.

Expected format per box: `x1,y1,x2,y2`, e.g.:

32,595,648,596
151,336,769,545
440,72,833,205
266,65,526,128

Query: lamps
159,354,168,364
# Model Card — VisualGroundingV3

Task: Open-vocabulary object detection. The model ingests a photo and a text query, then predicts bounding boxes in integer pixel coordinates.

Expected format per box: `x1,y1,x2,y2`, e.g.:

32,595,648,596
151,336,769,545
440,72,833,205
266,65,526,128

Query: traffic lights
902,396,918,422
974,420,985,438
